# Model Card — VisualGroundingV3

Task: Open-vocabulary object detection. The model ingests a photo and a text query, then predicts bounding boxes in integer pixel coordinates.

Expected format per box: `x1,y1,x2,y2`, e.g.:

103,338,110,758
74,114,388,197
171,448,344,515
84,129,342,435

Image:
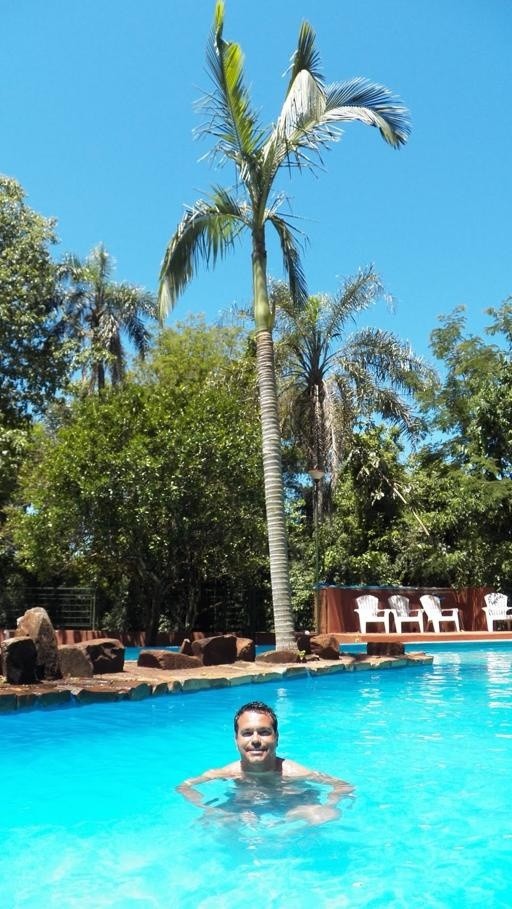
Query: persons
176,702,357,829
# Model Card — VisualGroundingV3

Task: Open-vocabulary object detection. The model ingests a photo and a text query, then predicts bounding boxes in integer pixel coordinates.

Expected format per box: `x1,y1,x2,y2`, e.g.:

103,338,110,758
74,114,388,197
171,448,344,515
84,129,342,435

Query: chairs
481,593,512,632
353,594,390,634
388,594,425,634
419,594,460,632
